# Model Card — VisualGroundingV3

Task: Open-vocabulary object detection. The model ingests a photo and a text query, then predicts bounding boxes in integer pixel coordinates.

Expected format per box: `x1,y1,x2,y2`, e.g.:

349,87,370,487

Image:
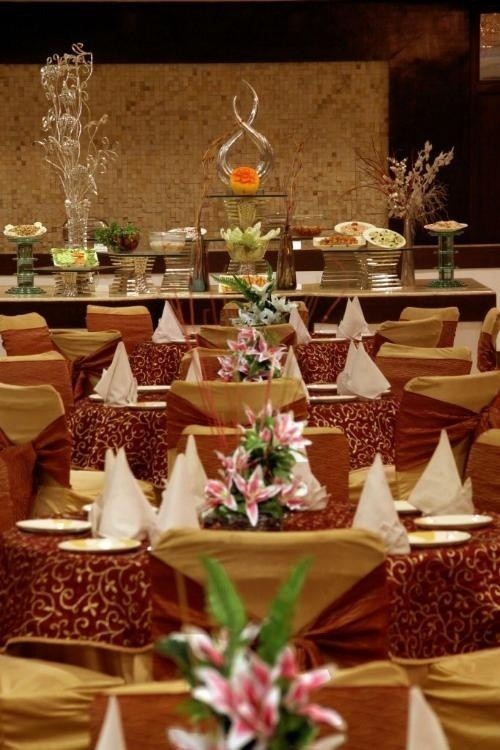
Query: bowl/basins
147,230,186,251
291,215,324,236
224,237,270,261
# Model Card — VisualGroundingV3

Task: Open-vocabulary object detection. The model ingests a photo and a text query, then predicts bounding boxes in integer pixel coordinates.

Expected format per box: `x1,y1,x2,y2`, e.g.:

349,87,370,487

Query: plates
309,395,357,403
115,399,168,410
361,333,376,338
51,247,99,271
409,530,472,546
172,339,197,344
394,501,423,511
312,220,407,250
88,393,104,402
16,517,93,531
414,515,493,529
310,337,347,343
4,225,47,238
423,221,468,232
169,225,208,241
306,383,338,390
314,330,338,335
137,385,173,392
82,502,160,516
58,539,141,554
